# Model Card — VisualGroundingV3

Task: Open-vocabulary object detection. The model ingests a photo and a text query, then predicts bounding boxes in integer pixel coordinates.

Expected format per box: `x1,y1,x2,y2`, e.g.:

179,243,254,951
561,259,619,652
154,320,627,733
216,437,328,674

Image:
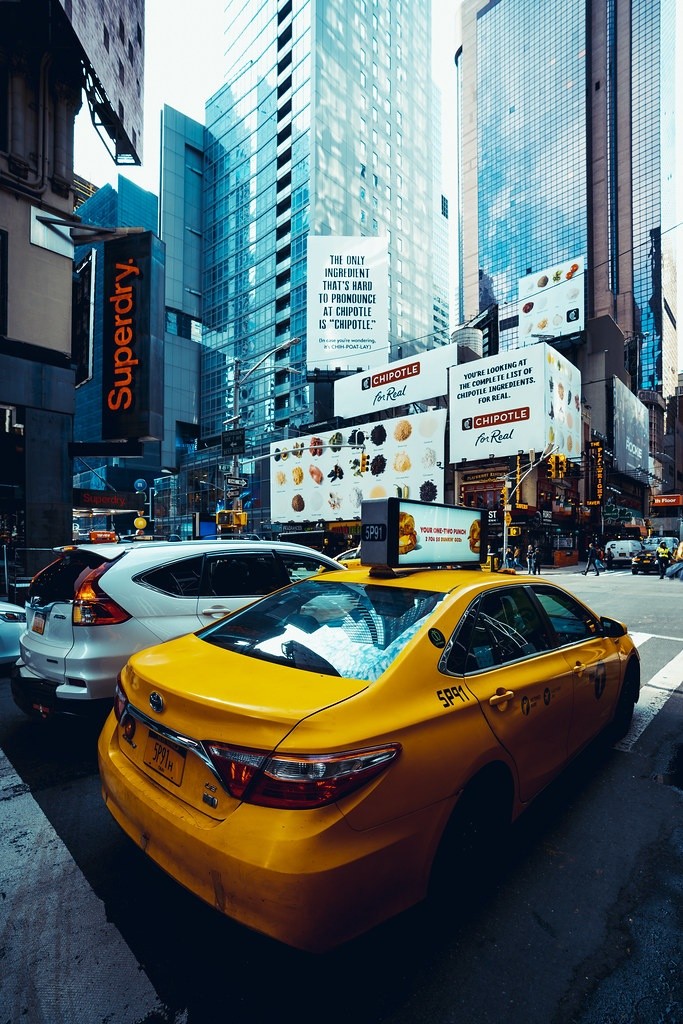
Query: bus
74,508,143,542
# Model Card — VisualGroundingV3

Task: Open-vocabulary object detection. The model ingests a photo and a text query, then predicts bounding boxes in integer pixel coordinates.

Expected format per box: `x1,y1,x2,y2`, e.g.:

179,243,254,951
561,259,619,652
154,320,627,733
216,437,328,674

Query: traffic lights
507,526,520,535
545,455,558,478
215,511,232,527
239,511,249,527
359,451,370,472
559,454,567,476
500,490,508,505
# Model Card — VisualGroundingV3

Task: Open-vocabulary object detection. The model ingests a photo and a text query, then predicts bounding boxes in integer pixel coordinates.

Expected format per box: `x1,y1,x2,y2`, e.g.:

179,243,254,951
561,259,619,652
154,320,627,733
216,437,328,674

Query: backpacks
599,550,604,559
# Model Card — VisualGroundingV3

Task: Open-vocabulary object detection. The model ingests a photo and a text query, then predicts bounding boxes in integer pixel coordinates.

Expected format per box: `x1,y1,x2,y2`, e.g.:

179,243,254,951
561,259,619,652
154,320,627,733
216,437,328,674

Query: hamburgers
469,519,481,553
399,512,418,554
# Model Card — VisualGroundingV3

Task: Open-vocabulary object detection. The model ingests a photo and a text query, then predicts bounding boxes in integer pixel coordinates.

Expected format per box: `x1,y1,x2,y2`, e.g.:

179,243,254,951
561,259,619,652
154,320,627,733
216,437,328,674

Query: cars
0,602,30,664
91,497,646,951
318,544,367,576
632,550,672,575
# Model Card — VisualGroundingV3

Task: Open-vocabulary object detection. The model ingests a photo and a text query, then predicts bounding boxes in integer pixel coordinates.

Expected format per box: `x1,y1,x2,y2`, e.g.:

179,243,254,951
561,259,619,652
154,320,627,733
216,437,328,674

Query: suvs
15,541,366,724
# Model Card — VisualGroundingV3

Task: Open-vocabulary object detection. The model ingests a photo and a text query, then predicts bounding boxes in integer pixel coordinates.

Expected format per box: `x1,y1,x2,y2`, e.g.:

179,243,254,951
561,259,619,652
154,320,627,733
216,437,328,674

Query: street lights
233,336,304,537
200,480,227,509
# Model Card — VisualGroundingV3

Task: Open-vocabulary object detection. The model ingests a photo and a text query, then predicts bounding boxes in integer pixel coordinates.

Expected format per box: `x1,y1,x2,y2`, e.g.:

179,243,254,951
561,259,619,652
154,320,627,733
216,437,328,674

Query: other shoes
595,574,599,576
582,572,586,575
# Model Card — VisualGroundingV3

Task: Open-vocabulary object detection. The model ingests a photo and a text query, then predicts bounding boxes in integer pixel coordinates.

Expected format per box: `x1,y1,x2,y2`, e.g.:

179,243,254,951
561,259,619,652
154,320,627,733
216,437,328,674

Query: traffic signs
226,476,249,489
225,488,241,498
221,428,247,457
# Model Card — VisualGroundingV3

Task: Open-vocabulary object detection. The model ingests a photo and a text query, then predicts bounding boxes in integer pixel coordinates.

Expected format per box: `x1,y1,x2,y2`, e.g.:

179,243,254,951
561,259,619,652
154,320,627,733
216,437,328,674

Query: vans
643,537,682,550
603,540,639,566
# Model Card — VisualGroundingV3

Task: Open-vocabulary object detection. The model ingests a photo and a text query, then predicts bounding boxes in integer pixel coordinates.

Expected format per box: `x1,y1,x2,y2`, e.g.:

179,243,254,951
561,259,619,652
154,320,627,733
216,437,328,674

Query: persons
604,547,615,572
532,548,541,575
512,545,524,570
526,544,534,575
484,544,493,563
655,541,675,579
582,543,599,576
504,547,513,569
320,538,336,561
593,545,606,572
